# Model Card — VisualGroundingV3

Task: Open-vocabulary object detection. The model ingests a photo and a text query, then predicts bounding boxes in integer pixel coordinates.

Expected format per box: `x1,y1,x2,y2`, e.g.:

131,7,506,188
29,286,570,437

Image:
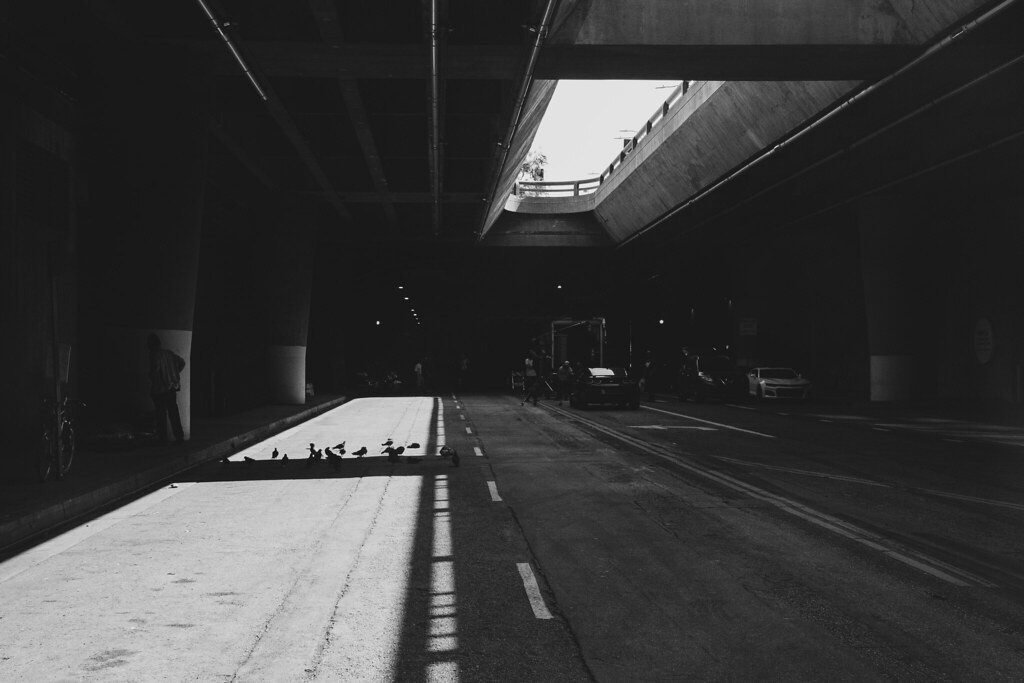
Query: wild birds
243,438,461,468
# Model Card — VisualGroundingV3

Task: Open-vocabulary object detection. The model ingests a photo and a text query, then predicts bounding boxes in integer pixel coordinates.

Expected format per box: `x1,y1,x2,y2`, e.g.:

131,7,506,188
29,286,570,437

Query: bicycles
40,398,87,483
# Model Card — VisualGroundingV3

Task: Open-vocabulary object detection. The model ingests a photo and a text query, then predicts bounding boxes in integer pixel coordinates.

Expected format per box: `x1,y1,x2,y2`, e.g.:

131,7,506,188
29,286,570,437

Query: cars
569,366,640,410
746,367,813,402
682,373,732,404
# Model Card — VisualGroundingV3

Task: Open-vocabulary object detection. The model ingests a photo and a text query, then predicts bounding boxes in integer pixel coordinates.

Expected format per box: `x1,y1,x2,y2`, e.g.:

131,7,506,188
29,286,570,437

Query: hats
680,345,688,348
562,360,570,365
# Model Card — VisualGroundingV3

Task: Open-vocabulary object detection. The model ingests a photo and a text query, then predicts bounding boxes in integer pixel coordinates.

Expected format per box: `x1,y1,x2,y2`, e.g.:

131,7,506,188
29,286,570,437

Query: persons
147,333,186,446
414,359,423,386
557,360,592,405
523,359,538,407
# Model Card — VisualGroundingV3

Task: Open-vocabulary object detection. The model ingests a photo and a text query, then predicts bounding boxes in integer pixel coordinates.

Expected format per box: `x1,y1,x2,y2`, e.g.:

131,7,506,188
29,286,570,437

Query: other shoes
523,398,529,402
155,436,168,444
558,403,561,405
533,399,537,405
173,439,185,446
